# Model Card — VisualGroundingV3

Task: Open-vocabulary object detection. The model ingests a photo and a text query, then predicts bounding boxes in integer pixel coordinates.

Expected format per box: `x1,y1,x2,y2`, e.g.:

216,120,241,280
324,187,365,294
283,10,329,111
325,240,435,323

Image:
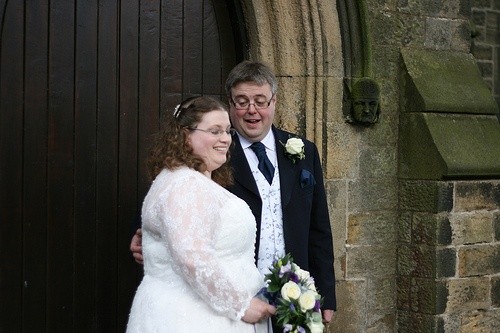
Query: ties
249,141,275,186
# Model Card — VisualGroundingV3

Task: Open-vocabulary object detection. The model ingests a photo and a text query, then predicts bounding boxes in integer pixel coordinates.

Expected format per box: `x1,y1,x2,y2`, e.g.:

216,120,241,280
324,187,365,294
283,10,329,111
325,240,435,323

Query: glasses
230,92,275,109
188,126,236,139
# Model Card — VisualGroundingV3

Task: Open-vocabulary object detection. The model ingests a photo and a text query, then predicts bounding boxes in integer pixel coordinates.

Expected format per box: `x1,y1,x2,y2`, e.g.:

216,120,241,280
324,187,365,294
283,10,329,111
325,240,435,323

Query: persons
129,59,337,333
125,94,279,333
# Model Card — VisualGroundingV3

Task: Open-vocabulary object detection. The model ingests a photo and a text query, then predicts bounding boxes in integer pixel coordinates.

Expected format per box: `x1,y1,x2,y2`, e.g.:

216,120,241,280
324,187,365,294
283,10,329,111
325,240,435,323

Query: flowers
278,133,306,165
263,252,325,333
